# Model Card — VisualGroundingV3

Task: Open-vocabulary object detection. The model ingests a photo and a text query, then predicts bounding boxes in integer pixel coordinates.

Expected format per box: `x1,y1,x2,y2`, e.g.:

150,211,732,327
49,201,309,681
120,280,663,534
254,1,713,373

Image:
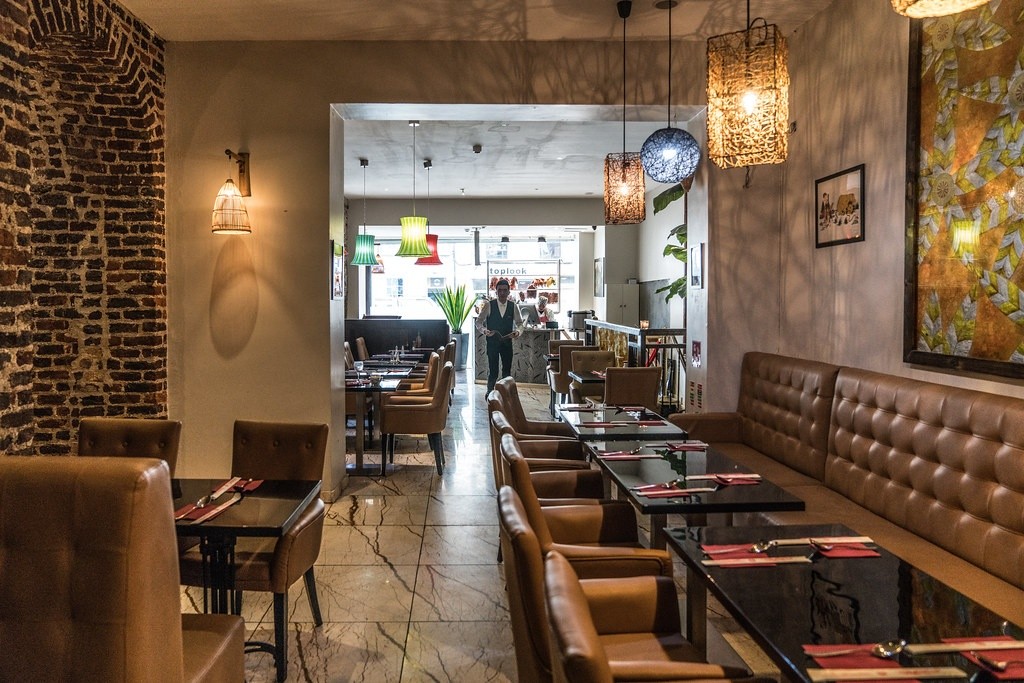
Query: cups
370,373,383,386
640,320,649,329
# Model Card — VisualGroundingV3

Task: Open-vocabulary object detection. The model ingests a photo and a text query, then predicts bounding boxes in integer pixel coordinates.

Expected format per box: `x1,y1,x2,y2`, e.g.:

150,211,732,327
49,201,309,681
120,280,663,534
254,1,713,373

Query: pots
567,309,595,332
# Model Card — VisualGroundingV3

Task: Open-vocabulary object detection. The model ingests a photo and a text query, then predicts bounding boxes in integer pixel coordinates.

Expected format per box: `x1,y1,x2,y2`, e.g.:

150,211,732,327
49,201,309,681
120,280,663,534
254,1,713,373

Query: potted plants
431,285,479,372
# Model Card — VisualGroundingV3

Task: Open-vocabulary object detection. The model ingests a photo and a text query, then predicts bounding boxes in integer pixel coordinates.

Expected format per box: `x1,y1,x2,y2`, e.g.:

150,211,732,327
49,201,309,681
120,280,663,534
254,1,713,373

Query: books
489,330,516,340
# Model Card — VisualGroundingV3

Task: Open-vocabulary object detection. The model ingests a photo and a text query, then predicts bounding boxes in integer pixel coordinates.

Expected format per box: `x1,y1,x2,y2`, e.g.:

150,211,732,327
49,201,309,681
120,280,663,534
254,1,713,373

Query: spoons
702,538,771,554
970,650,1024,671
174,495,211,521
714,474,763,483
810,539,878,551
803,639,907,658
233,478,252,492
628,478,680,489
666,442,709,449
598,447,643,455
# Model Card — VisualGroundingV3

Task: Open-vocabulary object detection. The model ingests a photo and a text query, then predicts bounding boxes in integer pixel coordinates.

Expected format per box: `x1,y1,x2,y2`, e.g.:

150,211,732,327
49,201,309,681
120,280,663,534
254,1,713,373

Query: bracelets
516,331,519,337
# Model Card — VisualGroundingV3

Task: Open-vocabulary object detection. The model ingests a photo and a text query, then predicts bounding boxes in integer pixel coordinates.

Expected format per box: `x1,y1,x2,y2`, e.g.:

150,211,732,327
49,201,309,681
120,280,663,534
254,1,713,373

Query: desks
568,369,605,403
542,353,559,361
345,367,412,451
583,440,806,550
171,474,323,618
663,522,1024,683
370,354,425,360
553,403,689,462
344,378,401,477
362,360,420,369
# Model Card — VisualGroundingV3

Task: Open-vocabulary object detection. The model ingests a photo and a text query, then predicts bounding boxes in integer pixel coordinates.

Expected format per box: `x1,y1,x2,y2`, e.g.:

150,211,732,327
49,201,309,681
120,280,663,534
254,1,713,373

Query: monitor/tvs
516,304,541,331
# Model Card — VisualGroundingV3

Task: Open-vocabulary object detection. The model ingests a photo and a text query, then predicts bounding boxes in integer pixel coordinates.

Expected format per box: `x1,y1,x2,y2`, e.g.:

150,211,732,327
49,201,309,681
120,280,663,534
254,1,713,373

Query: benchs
669,352,1024,629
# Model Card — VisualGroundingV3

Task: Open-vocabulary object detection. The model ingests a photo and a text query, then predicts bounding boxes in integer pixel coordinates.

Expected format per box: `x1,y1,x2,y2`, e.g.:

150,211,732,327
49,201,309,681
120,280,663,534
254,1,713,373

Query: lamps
395,120,431,258
350,158,379,267
537,237,546,242
603,0,646,226
210,148,252,235
890,0,990,19
639,0,701,183
371,243,385,274
706,0,790,170
501,236,510,242
415,162,443,265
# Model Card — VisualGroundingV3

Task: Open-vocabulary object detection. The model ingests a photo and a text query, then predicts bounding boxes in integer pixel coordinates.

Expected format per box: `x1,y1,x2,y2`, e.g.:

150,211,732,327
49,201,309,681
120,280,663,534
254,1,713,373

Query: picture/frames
902,0,1024,381
332,239,344,302
690,242,702,290
814,163,865,248
593,257,605,297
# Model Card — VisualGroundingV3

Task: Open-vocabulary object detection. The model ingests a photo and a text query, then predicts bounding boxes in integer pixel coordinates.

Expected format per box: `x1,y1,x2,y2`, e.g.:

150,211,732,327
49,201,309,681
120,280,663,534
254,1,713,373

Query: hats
538,296,547,306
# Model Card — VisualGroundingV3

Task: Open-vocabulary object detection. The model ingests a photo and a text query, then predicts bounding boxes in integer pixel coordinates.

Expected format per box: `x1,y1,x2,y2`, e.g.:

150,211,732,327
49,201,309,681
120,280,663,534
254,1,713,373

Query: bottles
389,331,420,364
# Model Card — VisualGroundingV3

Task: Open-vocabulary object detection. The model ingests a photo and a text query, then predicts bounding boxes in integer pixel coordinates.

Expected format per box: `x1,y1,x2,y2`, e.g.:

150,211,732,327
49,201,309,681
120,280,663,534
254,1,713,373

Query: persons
476,280,525,402
530,296,554,329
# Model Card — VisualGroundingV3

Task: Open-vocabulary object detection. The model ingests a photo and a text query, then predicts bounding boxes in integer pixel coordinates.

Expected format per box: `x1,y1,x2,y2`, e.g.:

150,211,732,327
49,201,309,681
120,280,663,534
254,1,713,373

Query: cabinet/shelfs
486,259,560,315
604,283,640,328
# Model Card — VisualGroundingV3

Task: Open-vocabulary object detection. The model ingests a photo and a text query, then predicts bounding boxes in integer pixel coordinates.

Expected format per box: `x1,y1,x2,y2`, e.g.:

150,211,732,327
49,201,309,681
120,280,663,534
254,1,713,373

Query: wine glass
354,361,363,385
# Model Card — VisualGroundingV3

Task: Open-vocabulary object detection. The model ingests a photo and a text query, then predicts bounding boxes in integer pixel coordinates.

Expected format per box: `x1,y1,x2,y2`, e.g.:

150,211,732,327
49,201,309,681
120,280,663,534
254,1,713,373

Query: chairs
356,336,369,361
344,341,354,368
177,420,329,683
486,339,778,683
77,418,182,477
380,337,457,476
0,456,247,683
